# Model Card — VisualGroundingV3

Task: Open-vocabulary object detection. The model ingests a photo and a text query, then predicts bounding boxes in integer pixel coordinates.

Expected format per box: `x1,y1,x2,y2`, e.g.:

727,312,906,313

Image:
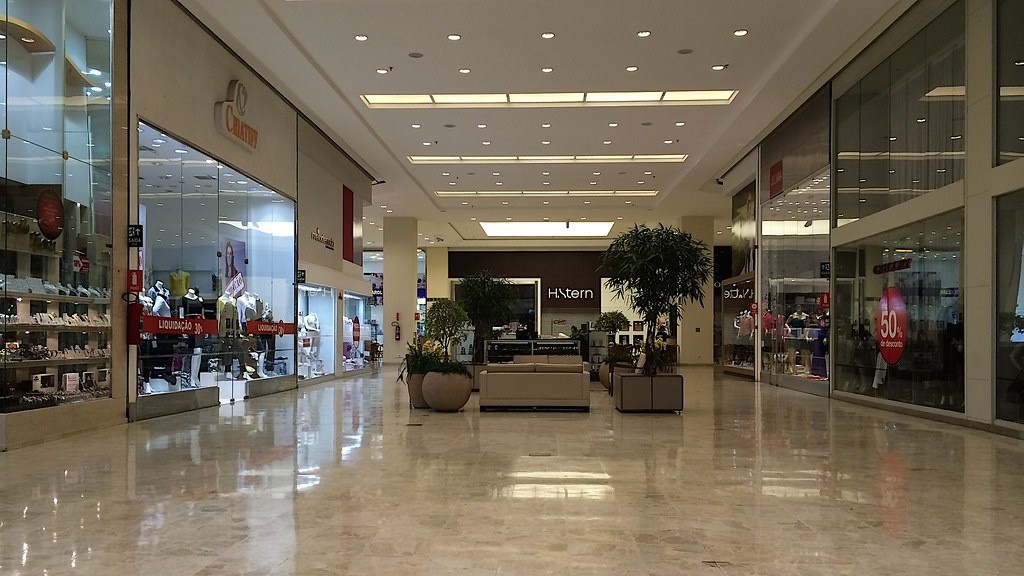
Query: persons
137,265,278,395
729,304,828,381
224,242,241,278
304,312,320,353
655,325,671,342
570,325,580,340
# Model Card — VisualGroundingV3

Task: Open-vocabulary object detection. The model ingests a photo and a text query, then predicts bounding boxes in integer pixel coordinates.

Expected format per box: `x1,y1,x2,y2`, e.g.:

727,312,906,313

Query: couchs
508,354,582,364
479,362,591,412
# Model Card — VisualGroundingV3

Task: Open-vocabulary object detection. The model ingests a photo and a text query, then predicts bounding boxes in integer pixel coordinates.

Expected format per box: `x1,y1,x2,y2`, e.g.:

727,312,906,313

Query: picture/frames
588,321,643,347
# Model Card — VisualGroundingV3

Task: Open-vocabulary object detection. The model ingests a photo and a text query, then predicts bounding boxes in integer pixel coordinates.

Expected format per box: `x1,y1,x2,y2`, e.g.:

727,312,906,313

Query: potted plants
395,341,441,408
592,309,632,389
421,298,473,412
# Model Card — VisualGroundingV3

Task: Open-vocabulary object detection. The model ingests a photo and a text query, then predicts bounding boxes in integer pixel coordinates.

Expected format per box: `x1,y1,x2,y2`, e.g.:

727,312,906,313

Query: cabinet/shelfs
137,298,218,365
767,292,830,322
0,289,113,418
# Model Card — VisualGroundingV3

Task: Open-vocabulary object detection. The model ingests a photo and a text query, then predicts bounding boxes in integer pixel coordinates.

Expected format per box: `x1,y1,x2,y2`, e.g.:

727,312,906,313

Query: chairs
608,353,646,397
370,343,383,362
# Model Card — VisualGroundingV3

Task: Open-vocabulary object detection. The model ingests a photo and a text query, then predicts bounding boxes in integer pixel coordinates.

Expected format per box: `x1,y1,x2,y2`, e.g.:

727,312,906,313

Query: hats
659,325,666,330
